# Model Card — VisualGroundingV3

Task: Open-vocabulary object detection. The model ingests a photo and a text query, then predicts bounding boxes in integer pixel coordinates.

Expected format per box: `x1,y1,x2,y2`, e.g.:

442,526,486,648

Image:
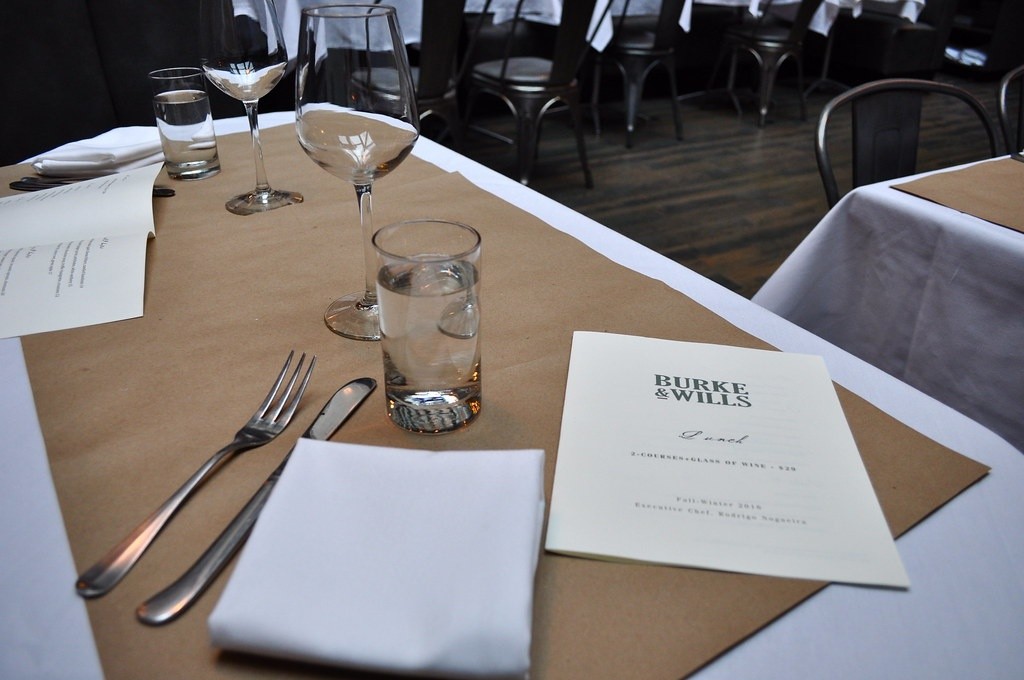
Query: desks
0,99,1024,680
748,152,1024,454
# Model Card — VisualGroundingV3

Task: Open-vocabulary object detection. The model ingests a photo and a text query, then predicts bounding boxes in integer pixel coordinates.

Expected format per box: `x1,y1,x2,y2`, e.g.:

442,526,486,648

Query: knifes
134,376,378,628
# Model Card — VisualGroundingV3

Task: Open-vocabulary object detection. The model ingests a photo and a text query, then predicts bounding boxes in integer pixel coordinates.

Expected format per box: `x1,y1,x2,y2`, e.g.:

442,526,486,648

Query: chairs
348,0,1024,215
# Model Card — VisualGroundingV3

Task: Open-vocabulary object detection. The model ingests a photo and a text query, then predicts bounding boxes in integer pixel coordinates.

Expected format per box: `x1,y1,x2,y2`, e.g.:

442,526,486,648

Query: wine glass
194,0,304,215
292,4,422,340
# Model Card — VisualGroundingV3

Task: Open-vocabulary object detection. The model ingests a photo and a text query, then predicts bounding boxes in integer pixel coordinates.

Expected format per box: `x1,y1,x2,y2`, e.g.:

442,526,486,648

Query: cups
147,66,221,182
372,218,485,436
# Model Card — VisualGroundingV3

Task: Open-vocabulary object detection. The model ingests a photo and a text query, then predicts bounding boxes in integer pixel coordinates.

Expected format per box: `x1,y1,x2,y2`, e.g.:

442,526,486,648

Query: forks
73,350,319,597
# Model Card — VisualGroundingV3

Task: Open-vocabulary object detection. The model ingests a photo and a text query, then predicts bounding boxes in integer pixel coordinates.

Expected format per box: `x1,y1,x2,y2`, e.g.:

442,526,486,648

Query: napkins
33,126,167,179
205,437,546,680
